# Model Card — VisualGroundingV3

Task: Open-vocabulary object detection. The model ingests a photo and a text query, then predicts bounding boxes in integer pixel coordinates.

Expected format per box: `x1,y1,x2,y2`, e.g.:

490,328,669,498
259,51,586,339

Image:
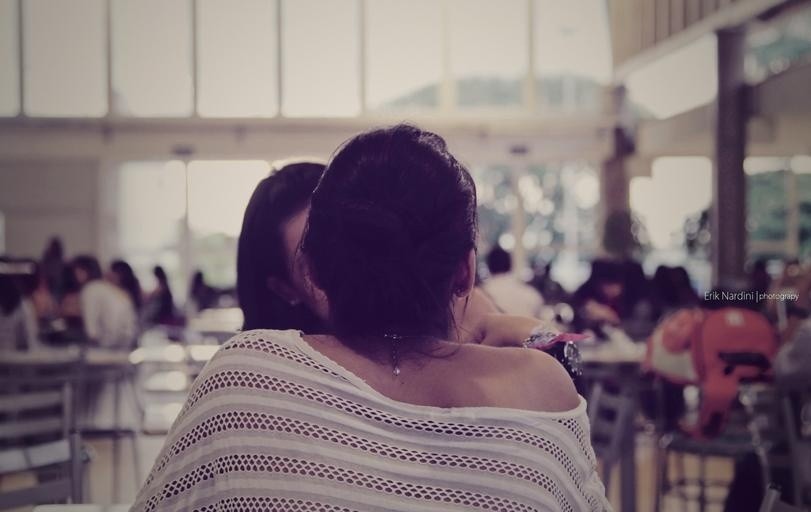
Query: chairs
1,279,239,507
557,331,807,512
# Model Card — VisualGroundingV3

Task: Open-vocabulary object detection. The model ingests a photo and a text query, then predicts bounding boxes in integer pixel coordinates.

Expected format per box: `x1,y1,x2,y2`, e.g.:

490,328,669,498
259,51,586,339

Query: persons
0,125,811,510
